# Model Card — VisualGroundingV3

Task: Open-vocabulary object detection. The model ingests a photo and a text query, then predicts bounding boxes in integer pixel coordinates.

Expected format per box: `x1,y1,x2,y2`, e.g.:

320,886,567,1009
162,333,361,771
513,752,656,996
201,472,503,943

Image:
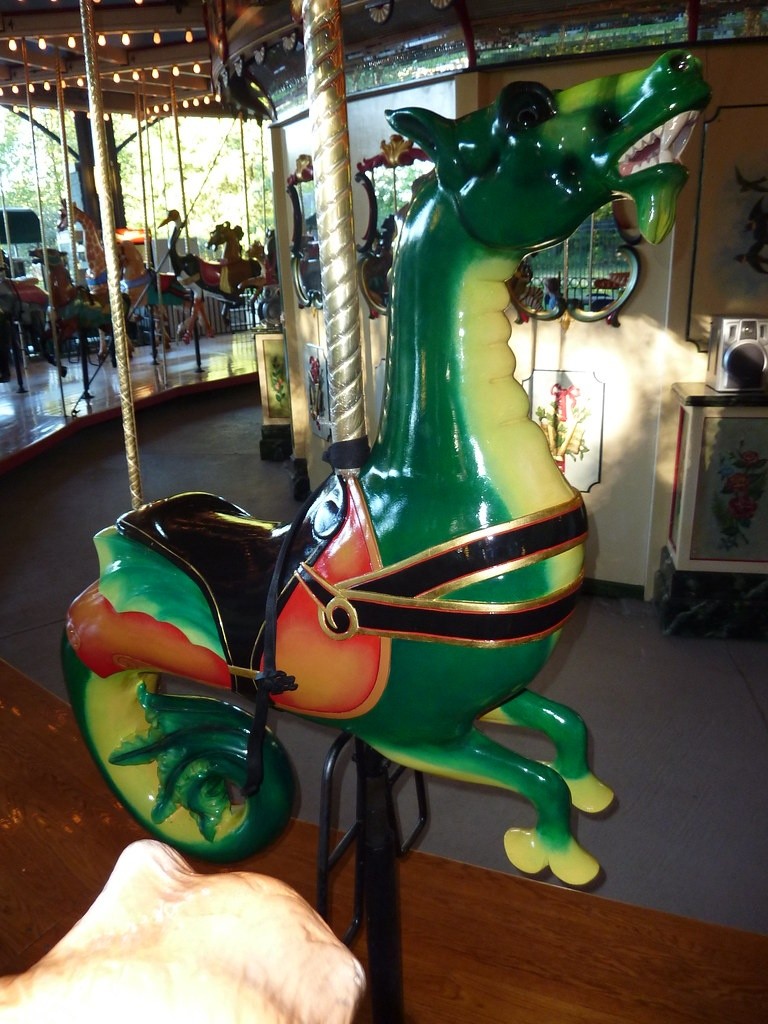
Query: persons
1,835,371,1024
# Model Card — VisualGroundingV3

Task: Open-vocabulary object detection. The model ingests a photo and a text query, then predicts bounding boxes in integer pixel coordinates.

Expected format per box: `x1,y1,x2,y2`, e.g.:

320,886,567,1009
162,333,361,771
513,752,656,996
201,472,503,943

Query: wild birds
159,209,261,342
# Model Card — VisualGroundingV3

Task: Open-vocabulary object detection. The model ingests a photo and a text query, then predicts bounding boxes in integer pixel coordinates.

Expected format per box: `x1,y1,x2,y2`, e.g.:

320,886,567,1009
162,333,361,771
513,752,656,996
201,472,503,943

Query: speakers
704,314,768,392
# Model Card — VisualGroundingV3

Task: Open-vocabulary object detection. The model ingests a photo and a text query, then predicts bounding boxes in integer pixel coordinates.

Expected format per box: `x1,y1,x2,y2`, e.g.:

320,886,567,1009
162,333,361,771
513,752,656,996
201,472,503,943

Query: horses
0,223,262,378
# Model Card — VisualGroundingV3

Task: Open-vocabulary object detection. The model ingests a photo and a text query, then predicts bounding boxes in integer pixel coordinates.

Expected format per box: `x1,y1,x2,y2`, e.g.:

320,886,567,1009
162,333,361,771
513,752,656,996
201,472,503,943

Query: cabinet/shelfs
656,382,768,631
